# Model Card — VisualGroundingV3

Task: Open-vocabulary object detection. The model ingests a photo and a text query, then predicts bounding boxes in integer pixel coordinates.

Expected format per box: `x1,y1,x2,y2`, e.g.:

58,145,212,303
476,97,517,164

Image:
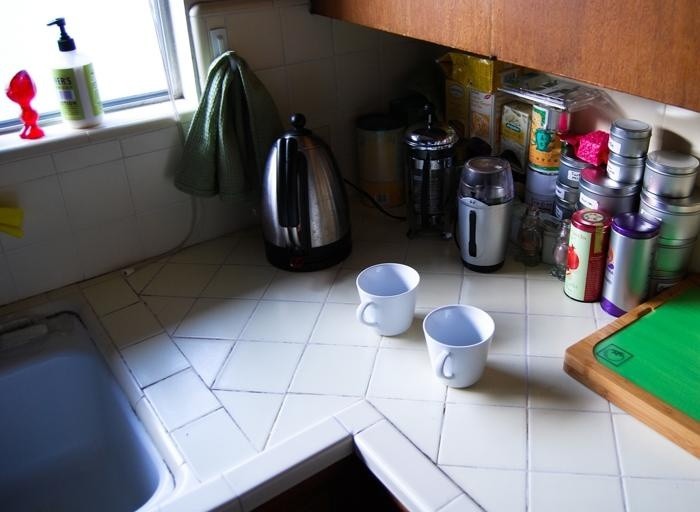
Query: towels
172,50,284,204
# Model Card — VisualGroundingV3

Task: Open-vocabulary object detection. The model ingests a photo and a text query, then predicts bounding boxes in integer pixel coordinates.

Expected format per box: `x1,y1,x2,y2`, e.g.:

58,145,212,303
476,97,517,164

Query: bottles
551,218,570,281
522,207,543,267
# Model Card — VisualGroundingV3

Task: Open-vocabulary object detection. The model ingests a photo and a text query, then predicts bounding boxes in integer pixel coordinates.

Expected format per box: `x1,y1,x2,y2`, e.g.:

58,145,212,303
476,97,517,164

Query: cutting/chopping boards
564,272,699,461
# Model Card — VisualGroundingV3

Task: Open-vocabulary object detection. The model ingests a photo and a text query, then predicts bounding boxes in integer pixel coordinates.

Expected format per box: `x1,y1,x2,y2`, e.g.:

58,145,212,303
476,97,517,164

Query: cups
600,212,662,319
422,304,496,390
457,156,515,274
355,262,421,338
354,111,407,210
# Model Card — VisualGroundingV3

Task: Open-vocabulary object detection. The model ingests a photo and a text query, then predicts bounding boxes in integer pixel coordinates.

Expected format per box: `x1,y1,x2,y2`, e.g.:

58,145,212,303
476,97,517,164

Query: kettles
260,111,354,274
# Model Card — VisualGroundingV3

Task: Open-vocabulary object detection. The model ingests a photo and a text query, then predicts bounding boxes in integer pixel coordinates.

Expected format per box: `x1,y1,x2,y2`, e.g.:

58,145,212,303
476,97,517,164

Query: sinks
1,309,176,511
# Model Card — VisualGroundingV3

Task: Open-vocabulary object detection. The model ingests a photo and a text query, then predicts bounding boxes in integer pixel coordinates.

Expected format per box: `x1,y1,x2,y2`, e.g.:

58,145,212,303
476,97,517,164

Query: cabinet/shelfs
308,0,700,116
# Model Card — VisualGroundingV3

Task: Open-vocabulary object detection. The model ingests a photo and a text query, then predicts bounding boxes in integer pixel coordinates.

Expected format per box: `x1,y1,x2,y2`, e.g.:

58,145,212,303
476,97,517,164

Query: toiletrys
45,16,105,128
